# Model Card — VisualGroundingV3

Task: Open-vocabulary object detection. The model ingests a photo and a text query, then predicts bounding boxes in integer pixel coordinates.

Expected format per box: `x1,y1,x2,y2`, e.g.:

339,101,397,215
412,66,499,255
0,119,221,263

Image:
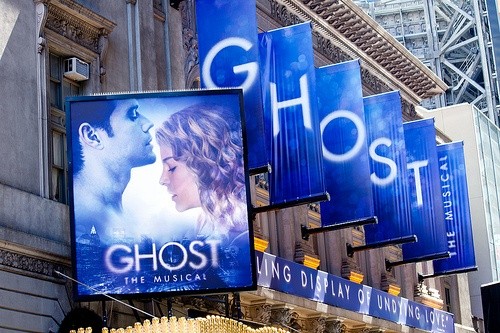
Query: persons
154,102,248,240
71,99,156,238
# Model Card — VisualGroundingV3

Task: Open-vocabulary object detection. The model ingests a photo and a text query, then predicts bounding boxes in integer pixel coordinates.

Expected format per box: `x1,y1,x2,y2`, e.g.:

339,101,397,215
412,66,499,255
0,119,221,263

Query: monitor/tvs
66,88,258,302
